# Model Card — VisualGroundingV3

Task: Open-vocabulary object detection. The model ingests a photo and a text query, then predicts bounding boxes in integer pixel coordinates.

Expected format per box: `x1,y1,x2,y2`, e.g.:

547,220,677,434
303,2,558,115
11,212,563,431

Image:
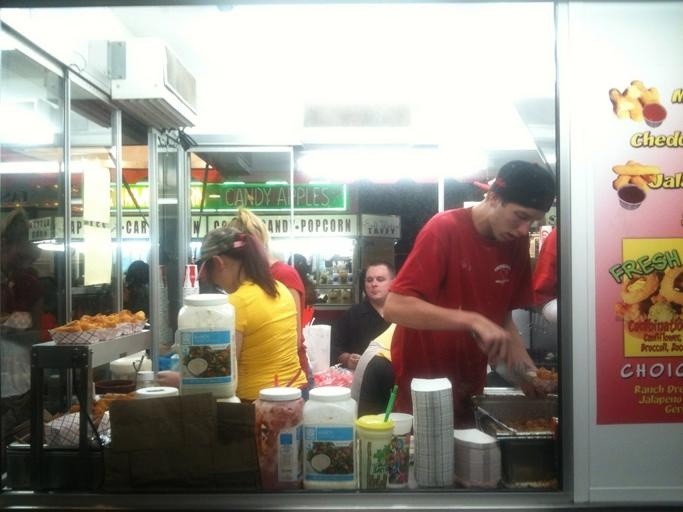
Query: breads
53,309,146,333
68,392,138,420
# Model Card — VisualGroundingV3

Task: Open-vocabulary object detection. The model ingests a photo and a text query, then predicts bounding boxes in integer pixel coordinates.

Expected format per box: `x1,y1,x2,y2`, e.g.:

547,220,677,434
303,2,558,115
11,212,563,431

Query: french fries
610,80,660,123
611,160,658,192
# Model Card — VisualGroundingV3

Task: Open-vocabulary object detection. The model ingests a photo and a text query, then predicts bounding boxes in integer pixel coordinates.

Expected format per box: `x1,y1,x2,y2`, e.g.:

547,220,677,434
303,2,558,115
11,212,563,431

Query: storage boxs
109,392,259,488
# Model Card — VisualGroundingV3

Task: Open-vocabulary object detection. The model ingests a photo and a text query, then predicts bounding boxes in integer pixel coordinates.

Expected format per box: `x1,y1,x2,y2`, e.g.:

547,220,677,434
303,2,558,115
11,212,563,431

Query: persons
332,261,396,372
351,322,397,420
156,226,308,407
0,230,44,430
120,260,150,328
288,253,316,304
529,226,558,325
383,160,556,434
225,208,314,402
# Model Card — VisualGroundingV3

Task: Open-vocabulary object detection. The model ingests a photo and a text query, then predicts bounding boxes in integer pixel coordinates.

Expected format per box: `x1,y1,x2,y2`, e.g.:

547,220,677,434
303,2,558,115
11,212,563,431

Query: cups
356,413,413,491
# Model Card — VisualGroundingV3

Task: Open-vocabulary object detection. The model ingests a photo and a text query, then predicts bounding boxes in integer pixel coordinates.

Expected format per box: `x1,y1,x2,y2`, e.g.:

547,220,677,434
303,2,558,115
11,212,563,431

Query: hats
472,160,557,213
193,226,248,264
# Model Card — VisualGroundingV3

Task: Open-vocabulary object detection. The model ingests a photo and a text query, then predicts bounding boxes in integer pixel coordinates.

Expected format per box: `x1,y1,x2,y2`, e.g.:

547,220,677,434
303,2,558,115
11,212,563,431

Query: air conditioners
109,33,201,137
